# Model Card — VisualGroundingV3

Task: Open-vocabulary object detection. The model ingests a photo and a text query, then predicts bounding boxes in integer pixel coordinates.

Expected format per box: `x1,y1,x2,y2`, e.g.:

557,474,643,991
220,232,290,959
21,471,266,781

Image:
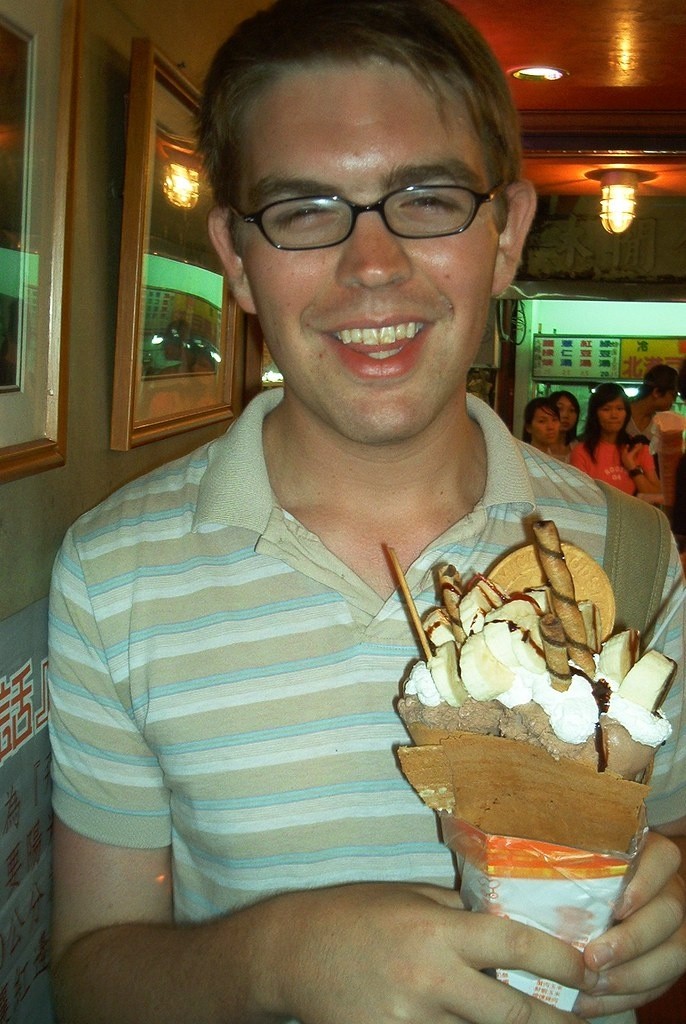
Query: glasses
225,175,504,251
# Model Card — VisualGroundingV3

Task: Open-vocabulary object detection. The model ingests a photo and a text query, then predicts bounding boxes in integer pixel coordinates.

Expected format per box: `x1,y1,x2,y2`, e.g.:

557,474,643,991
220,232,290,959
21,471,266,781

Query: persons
523,362,686,574
49,0,685,1024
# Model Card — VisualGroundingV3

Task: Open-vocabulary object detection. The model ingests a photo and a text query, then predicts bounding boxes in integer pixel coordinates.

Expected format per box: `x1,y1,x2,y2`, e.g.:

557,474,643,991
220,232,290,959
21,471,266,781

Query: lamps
598,170,639,236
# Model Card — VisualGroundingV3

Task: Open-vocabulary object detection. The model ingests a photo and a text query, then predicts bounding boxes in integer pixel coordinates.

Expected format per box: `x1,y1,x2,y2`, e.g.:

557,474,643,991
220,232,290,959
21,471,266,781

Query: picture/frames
109,37,247,451
1,0,86,484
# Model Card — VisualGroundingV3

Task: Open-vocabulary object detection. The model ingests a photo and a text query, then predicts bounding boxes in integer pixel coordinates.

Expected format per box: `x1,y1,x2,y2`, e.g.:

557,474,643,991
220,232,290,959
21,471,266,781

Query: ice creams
382,517,677,863
